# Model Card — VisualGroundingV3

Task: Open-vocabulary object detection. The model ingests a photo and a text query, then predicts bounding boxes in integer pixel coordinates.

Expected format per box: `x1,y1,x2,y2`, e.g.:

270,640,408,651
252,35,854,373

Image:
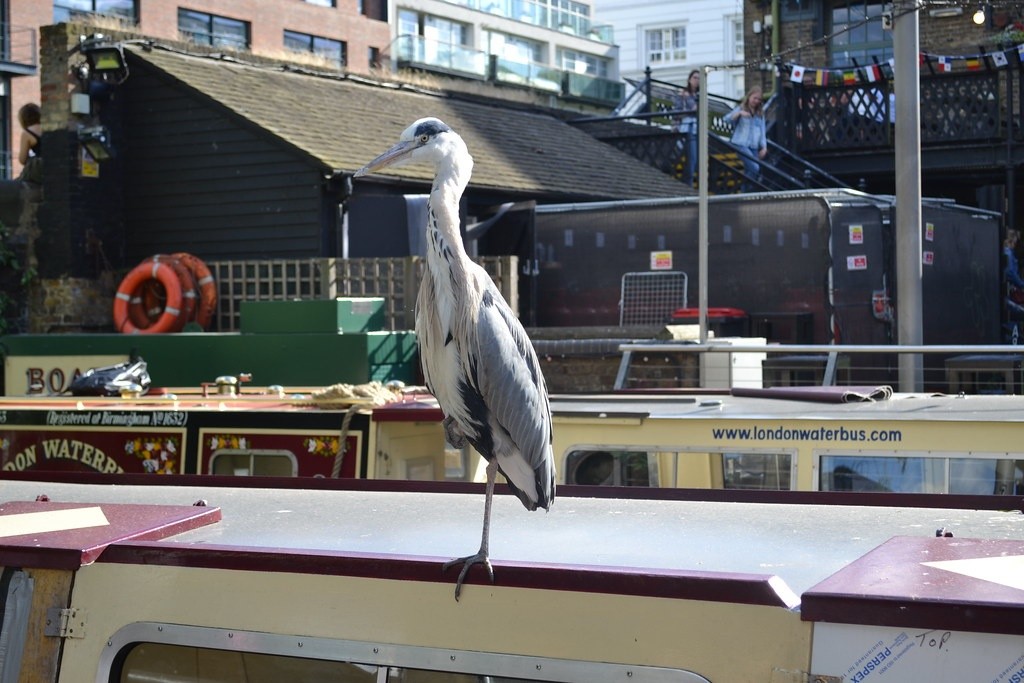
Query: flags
992,52,1008,67
938,57,952,71
965,57,979,70
816,59,894,86
1017,44,1024,61
790,66,805,83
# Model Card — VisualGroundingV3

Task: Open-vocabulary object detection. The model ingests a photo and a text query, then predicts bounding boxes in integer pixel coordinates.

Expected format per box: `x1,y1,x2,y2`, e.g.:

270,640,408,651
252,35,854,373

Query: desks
945,355,1024,394
748,310,815,385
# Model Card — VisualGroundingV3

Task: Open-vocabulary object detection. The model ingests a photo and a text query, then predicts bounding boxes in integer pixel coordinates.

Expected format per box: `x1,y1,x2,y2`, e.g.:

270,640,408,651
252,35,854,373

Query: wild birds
351,117,557,601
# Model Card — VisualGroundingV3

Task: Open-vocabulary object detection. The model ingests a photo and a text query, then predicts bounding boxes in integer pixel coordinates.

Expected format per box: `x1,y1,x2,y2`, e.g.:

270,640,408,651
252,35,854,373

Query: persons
19,103,42,166
723,85,766,192
1004,229,1024,288
670,70,700,186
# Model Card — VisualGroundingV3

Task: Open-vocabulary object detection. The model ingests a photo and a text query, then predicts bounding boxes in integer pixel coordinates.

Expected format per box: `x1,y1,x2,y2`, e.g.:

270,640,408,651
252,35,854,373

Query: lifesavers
127,253,196,332
113,262,183,334
145,252,216,331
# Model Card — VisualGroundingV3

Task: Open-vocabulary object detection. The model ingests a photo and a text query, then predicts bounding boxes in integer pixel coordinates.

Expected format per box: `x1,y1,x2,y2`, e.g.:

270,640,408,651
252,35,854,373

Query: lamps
85,46,129,85
76,125,118,161
972,3,992,30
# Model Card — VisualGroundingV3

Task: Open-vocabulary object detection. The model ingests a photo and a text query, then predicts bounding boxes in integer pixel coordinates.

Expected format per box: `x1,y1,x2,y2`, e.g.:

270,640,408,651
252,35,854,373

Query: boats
0,66,1024,683
